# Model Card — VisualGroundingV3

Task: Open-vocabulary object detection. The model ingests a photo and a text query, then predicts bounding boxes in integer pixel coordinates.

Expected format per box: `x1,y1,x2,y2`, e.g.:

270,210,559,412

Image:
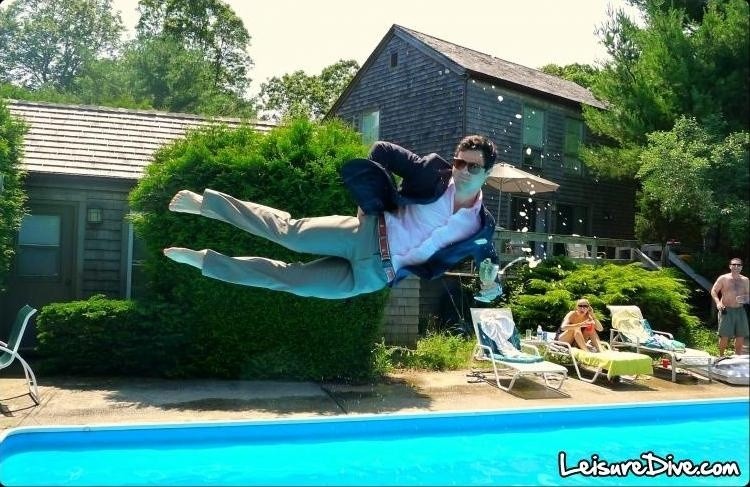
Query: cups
586,319,593,331
653,361,659,369
663,359,668,368
722,305,728,315
525,329,531,341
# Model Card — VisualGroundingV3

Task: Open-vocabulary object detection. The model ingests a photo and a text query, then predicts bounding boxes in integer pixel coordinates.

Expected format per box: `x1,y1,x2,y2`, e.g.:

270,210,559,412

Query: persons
163,135,497,300
554,299,604,353
711,258,750,357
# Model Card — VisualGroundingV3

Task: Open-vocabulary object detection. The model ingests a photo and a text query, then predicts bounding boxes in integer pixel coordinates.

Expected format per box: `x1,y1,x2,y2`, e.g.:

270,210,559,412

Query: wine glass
472,261,500,303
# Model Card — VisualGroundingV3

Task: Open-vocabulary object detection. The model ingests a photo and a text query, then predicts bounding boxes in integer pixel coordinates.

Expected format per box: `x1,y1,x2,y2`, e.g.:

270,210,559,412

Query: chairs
564,234,606,259
470,305,750,392
0,304,40,405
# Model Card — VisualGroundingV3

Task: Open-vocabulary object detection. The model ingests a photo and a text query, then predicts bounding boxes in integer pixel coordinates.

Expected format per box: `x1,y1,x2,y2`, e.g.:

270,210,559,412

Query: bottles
537,324,543,343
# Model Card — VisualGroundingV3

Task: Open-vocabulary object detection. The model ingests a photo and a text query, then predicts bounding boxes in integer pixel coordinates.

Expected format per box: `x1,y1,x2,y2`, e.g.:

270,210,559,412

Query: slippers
466,372,486,378
467,378,486,383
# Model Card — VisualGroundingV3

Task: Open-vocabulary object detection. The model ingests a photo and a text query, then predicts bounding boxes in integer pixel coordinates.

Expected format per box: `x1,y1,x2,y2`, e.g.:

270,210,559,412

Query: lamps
86,205,104,224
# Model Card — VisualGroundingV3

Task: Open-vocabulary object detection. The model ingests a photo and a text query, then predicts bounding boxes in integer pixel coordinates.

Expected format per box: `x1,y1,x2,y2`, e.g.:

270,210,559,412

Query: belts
379,213,397,284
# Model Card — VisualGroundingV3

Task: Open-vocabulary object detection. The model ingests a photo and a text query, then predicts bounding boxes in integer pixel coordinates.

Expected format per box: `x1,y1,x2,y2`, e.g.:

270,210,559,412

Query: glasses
578,304,588,308
730,263,742,266
453,156,487,174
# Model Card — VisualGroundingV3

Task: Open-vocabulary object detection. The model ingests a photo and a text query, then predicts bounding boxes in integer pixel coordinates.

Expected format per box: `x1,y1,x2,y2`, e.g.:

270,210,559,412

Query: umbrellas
485,161,561,226
